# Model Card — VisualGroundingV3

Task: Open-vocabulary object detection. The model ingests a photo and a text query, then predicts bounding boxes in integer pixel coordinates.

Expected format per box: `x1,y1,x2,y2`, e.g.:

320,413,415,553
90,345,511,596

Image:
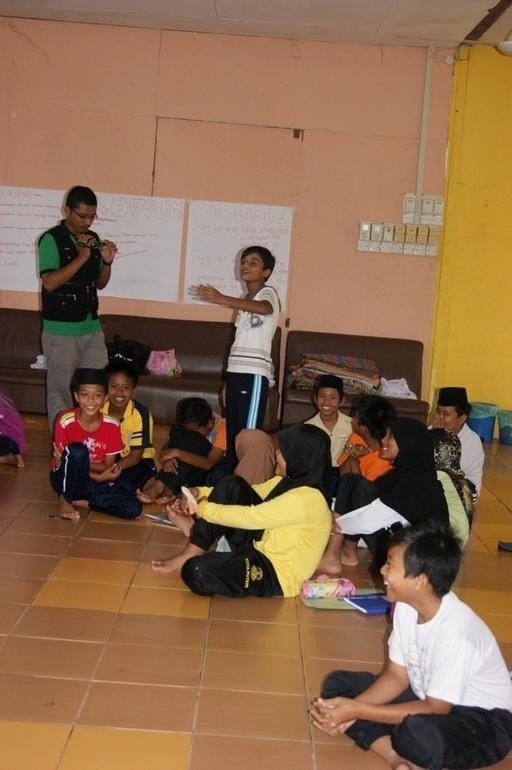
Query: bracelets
101,259,113,266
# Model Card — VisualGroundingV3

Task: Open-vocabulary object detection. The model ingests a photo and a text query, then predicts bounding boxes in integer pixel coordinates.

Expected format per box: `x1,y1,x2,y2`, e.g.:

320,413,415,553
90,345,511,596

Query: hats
438,386,468,406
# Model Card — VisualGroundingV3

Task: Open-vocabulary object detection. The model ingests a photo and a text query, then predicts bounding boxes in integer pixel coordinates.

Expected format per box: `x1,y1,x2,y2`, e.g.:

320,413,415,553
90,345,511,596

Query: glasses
71,207,99,220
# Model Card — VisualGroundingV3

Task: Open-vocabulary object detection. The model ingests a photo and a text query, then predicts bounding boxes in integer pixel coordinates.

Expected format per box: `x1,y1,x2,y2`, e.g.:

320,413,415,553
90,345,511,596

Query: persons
307,523,512,769
0,389,26,470
188,247,280,461
34,187,118,431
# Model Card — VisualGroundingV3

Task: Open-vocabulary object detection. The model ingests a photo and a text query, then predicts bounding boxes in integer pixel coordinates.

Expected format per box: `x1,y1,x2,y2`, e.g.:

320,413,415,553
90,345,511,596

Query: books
344,598,393,616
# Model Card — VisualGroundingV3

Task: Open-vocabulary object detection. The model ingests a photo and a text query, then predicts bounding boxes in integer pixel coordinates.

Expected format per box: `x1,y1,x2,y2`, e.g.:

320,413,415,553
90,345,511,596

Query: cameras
90,240,105,249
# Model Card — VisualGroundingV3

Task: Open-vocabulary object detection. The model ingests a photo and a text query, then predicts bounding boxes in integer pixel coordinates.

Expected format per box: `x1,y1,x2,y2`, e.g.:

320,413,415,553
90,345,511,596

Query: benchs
93,312,282,434
282,329,431,432
1,304,49,418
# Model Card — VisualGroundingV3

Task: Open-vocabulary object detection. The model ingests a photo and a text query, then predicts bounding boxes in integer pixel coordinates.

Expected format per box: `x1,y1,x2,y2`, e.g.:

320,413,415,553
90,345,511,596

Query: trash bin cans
469,400,499,444
496,408,512,446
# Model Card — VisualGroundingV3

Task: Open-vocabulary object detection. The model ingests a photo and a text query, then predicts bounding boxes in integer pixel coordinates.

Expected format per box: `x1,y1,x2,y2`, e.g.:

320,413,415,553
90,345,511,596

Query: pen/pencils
48,514,73,521
